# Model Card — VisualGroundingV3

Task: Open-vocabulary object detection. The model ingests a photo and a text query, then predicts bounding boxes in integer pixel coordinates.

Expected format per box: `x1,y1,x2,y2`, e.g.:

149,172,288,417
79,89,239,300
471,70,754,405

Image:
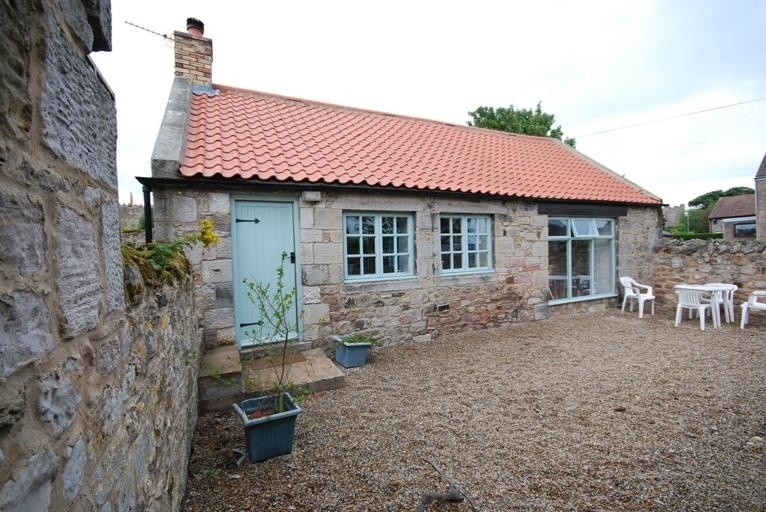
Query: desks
550,275,581,298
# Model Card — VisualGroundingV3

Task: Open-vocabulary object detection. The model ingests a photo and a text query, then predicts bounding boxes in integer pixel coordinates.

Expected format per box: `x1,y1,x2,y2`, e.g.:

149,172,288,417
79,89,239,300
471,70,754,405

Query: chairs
673,282,766,330
619,276,655,317
548,264,556,300
577,274,590,295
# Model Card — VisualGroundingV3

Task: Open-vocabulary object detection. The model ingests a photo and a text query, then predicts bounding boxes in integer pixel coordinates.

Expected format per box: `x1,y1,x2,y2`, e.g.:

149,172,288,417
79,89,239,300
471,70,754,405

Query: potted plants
331,329,379,367
179,249,324,462
